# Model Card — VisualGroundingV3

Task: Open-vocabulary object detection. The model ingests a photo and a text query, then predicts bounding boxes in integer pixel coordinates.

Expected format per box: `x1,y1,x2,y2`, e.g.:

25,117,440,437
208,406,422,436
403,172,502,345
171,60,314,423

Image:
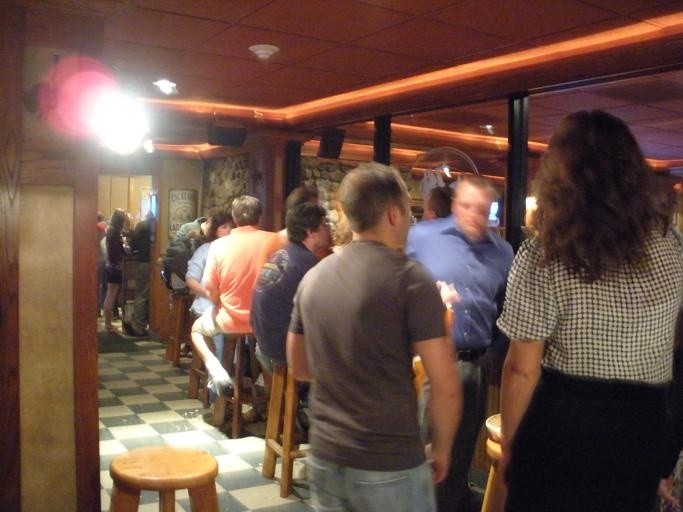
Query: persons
165,212,213,292
500,108,682,508
284,160,463,512
94,207,155,338
250,201,335,361
405,172,514,512
253,185,332,377
184,212,258,405
327,191,350,249
191,195,287,398
420,187,450,221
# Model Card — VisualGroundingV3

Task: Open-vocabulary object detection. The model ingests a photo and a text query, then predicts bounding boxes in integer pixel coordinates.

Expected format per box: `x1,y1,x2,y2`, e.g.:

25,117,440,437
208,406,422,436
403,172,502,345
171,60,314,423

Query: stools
262,356,311,498
212,333,262,439
482,412,514,511
109,447,219,512
165,292,195,367
186,316,210,409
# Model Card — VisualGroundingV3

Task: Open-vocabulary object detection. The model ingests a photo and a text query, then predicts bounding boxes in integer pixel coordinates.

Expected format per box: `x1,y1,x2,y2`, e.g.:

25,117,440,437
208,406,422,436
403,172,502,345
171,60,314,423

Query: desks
115,254,140,336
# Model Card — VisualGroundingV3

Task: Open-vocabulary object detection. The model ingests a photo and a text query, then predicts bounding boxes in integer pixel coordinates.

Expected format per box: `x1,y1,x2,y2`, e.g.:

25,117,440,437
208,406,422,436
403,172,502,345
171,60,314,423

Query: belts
454,346,488,363
